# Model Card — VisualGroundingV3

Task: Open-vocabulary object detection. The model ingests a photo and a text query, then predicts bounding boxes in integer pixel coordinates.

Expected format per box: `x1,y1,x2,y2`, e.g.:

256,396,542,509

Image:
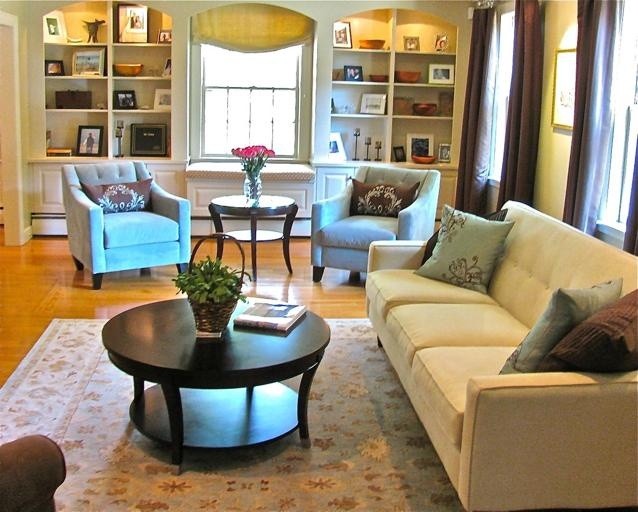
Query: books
234,301,307,332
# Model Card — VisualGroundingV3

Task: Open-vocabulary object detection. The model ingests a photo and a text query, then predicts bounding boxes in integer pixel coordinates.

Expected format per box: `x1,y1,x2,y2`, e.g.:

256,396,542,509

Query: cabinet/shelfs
309,0,474,220
28,0,188,236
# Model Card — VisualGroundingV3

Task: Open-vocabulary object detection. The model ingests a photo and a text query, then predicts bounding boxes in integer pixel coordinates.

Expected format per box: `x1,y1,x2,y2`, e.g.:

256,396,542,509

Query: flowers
231,145,276,198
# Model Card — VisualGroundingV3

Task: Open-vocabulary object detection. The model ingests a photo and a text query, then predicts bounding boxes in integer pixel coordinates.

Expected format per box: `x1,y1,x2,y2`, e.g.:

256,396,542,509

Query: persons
122,94,131,106
349,68,358,78
83,133,94,153
336,31,344,43
438,40,445,51
131,12,141,29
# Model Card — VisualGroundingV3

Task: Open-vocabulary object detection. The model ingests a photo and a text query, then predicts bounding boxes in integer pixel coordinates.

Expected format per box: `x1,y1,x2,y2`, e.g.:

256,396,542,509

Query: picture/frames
43,6,167,157
329,21,454,162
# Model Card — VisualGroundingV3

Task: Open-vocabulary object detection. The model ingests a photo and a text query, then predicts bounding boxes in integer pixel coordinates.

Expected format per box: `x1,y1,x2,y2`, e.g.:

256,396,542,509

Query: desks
208,194,298,281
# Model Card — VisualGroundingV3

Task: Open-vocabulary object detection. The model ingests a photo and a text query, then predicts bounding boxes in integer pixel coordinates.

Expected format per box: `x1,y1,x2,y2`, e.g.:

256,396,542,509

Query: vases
244,172,263,201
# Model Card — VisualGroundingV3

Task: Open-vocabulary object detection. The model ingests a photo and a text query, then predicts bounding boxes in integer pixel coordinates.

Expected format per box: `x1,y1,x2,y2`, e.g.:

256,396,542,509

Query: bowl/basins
395,71,422,82
369,75,387,82
413,155,436,164
359,40,385,49
413,103,437,116
114,63,144,76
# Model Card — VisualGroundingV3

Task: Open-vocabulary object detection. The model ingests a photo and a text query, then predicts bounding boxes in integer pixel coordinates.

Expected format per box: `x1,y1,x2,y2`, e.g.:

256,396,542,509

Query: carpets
0,318,465,512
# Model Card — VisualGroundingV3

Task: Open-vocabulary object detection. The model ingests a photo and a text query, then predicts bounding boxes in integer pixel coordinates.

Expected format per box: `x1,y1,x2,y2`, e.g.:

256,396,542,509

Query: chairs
311,165,441,280
62,160,191,289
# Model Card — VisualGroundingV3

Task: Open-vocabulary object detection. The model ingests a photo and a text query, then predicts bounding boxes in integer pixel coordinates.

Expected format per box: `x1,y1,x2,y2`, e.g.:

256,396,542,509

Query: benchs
186,162,315,237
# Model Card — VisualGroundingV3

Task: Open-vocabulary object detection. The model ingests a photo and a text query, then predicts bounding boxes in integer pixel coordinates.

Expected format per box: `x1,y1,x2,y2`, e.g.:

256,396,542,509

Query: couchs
366,198,638,512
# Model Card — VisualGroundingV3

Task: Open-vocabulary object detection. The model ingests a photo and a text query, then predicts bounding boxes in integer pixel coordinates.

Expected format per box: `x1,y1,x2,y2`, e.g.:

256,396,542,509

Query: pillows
350,178,420,216
81,178,153,214
412,203,515,294
421,208,507,265
498,277,625,373
537,290,638,373
